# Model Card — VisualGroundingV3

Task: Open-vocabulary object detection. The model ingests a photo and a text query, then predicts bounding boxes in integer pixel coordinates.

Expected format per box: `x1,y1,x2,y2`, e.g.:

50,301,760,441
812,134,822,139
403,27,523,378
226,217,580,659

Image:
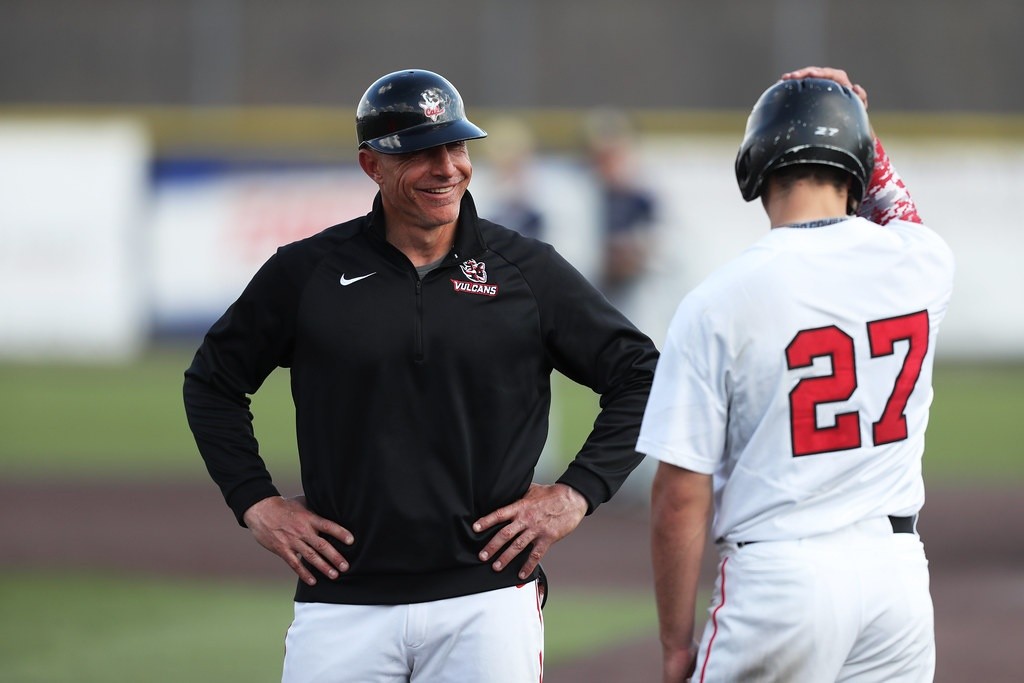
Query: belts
737,515,915,548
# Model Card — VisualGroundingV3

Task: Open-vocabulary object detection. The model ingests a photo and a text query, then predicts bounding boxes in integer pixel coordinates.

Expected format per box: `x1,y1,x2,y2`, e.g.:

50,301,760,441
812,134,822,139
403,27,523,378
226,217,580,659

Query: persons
475,112,548,245
574,107,675,353
181,67,661,683
633,67,959,683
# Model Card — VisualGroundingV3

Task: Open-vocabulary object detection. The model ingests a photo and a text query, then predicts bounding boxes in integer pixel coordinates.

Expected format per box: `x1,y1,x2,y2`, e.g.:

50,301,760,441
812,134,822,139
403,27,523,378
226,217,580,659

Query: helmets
734,77,874,215
356,69,487,154
